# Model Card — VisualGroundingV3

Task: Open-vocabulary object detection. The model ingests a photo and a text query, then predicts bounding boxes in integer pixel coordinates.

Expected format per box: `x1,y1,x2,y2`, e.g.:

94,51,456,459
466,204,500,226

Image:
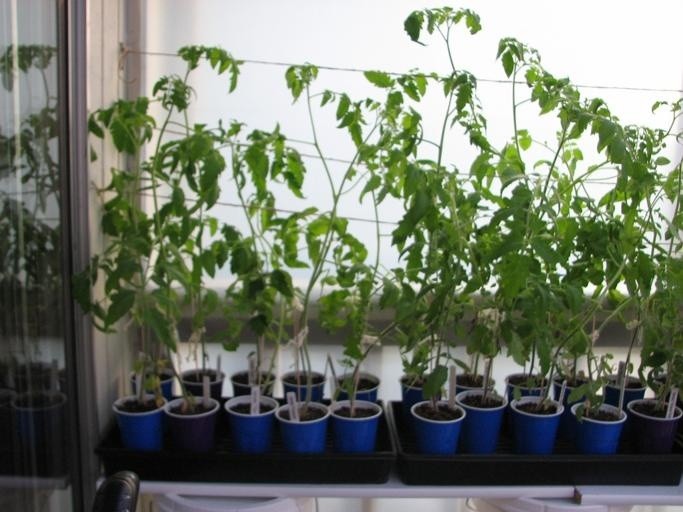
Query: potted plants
70,6,683,456
0,42,72,449
